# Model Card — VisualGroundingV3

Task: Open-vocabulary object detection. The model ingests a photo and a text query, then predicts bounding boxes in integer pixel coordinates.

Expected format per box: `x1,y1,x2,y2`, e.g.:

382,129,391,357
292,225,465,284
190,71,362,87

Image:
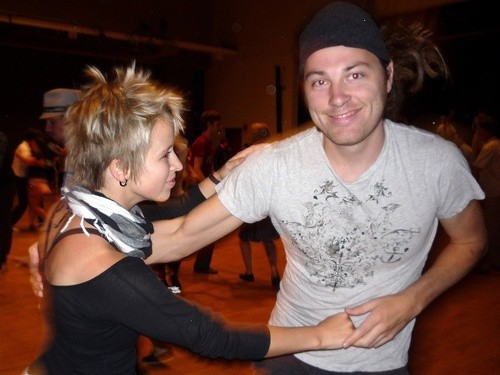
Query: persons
28,2,489,375
24,67,356,375
436,114,458,144
182,111,224,274
237,122,281,289
40,88,83,143
376,19,448,125
11,128,54,234
467,112,500,272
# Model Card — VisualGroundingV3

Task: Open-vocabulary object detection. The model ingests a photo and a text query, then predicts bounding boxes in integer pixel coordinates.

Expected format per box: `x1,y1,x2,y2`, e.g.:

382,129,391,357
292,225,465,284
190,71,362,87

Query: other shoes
193,265,218,273
141,346,173,364
271,276,281,289
167,271,181,291
240,273,254,281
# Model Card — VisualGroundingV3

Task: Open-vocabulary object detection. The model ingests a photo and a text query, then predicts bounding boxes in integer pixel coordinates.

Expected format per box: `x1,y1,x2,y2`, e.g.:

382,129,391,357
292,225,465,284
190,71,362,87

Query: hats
39,88,82,119
298,3,390,64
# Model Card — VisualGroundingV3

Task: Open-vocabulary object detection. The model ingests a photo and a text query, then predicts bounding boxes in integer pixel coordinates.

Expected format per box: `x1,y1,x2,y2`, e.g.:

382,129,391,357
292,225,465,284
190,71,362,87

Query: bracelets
208,173,221,185
